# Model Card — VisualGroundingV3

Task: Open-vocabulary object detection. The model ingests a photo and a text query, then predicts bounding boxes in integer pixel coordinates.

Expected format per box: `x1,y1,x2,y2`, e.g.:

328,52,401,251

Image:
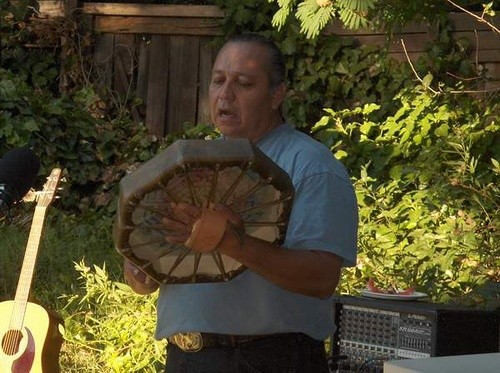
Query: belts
166,331,296,354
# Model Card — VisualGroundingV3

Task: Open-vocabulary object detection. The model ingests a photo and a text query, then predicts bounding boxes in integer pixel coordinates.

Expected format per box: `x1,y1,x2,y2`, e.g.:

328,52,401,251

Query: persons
124,34,359,372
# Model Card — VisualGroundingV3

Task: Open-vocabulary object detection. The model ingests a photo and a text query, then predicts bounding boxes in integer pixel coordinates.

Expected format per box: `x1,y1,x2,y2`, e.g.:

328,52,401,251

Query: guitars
0,168,65,373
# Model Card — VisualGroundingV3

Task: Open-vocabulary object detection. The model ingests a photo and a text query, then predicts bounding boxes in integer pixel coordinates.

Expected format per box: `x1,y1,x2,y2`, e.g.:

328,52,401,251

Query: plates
358,285,429,301
